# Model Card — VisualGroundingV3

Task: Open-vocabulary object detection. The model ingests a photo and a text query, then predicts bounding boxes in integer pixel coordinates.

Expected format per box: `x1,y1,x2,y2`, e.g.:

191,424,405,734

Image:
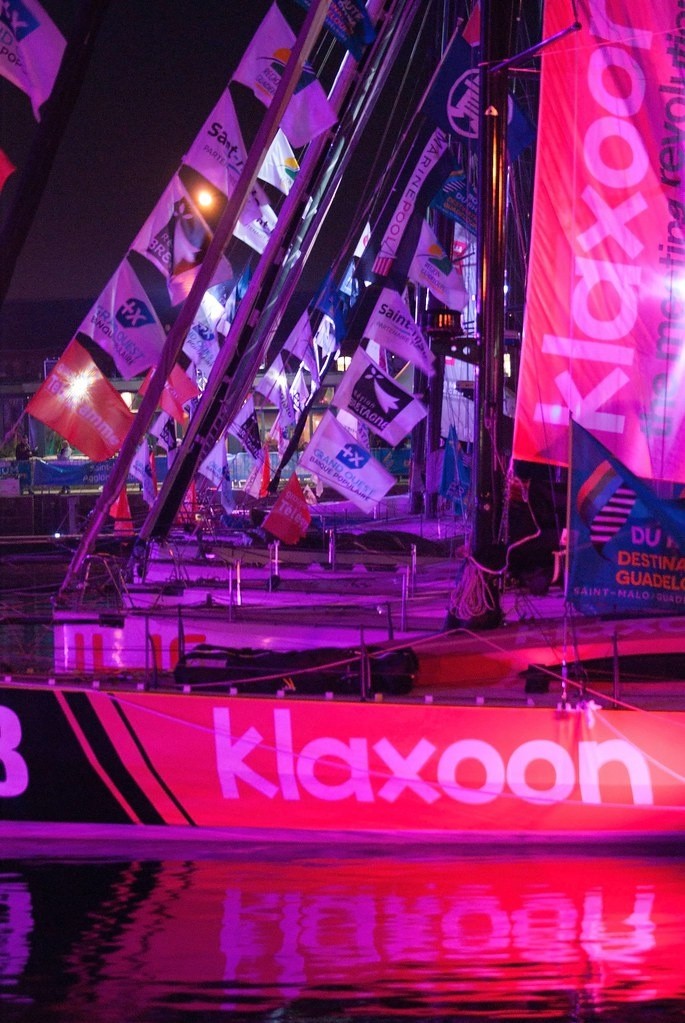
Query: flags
0,0,66,122
562,410,685,620
24,0,483,545
0,146,17,194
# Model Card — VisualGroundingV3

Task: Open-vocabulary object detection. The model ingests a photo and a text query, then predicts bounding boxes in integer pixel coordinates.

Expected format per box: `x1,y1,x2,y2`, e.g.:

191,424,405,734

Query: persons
57,441,73,494
16,435,39,495
138,445,154,491
176,438,183,447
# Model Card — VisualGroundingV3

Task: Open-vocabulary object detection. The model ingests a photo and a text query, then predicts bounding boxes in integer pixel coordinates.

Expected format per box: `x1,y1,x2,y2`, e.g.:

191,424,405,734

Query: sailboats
57,851,684,1022
0,0,685,853
0,855,685,1023
